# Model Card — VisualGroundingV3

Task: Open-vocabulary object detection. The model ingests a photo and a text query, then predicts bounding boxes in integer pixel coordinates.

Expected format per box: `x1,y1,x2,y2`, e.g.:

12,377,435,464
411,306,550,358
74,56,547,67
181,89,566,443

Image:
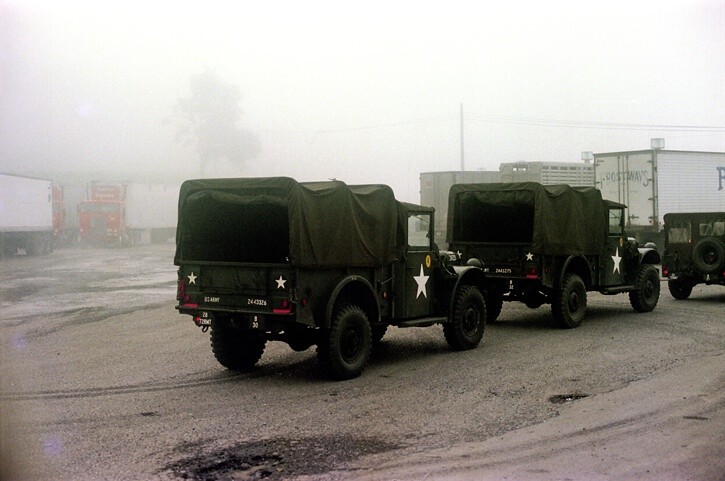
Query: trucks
175,177,491,378
445,181,661,328
0,174,180,256
593,148,725,257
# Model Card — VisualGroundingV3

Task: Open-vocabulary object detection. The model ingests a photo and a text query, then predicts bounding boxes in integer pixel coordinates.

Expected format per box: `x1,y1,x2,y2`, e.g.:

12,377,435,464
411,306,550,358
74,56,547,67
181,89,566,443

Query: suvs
662,212,725,300
